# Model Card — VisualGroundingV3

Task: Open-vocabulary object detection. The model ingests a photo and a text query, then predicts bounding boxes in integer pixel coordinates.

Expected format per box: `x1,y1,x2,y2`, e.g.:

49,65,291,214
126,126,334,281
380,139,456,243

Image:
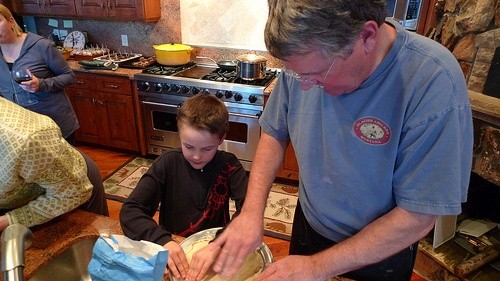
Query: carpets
101,156,299,242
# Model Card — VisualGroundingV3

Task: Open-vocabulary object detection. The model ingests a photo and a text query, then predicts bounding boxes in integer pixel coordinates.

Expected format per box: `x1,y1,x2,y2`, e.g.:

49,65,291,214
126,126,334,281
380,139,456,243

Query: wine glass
11,63,39,105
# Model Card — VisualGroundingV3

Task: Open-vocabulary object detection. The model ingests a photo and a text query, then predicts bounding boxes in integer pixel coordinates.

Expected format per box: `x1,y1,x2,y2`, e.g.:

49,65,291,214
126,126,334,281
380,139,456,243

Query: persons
209,0,474,281
0,97,110,232
119,94,249,281
0,5,80,146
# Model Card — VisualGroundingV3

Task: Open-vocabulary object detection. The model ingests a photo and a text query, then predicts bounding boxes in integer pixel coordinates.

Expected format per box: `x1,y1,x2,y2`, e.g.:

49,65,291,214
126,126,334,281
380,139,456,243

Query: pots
196,56,239,71
235,54,268,81
153,42,193,66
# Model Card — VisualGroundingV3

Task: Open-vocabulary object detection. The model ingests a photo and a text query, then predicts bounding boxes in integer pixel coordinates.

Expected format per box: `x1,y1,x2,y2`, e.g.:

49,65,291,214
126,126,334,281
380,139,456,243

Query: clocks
63,31,86,50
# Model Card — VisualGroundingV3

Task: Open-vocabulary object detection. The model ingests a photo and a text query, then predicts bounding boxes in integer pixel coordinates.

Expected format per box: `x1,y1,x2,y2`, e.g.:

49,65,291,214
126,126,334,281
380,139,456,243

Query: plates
63,30,85,51
92,52,142,62
166,226,274,281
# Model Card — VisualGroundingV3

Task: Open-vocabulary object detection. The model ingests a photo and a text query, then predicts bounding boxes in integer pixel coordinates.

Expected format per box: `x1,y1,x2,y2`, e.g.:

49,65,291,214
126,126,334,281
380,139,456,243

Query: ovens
138,92,261,172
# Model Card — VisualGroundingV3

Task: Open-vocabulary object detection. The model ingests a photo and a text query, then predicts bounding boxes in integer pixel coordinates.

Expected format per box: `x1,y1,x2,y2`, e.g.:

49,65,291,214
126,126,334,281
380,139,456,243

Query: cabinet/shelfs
74,0,161,22
12,0,77,19
66,72,139,152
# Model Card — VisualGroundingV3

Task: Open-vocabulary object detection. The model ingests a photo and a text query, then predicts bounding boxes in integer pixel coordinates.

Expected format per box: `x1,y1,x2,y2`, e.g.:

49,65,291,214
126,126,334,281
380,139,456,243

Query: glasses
279,59,336,89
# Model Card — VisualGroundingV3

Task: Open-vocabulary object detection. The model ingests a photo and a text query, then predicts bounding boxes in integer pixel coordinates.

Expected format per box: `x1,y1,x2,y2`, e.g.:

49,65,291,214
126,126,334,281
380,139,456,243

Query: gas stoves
135,61,281,111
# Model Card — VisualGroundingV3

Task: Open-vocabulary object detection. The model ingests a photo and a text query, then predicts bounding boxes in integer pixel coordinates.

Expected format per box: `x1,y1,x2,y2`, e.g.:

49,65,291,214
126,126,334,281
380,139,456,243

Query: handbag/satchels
456,217,500,272
